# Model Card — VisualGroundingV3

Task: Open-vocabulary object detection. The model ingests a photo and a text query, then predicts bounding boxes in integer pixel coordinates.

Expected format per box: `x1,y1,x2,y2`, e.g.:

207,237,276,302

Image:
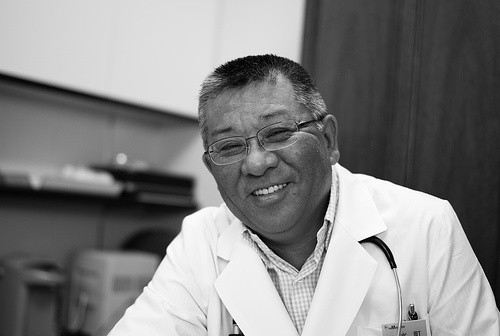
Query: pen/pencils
407,304,419,320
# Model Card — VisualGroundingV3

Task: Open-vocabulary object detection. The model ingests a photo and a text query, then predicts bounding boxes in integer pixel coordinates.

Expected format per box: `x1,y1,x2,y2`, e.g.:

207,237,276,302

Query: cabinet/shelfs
299,0,500,308
0,71,201,336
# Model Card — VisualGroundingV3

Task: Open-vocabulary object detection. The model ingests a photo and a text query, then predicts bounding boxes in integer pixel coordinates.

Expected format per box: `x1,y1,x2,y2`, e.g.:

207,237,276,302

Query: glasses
203,118,323,166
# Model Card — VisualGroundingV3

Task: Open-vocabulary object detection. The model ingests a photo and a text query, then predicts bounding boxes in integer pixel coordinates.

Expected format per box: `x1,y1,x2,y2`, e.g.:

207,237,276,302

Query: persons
106,54,500,336
